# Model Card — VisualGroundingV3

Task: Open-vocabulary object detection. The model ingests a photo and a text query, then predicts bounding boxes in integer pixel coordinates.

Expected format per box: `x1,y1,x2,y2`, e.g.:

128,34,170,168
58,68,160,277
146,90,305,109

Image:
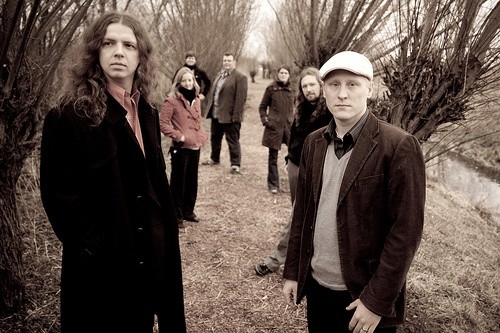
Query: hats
320,50,373,80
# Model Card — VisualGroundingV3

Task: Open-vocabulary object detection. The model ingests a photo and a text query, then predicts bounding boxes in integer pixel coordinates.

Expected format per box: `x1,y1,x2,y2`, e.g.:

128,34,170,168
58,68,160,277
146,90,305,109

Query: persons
202,54,247,173
40,13,186,332
282,50,425,333
159,67,208,225
250,62,273,83
258,65,299,194
171,52,212,119
253,67,334,275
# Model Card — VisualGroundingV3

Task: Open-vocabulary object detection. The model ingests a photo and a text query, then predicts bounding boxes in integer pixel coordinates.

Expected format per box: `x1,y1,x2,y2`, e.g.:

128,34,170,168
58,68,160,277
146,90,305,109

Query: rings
361,327,368,332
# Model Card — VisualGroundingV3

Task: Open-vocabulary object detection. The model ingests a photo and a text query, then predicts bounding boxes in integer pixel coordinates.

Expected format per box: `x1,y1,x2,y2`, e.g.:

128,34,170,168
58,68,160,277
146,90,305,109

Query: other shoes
270,186,279,193
202,159,213,165
193,216,199,222
230,165,240,174
178,222,185,227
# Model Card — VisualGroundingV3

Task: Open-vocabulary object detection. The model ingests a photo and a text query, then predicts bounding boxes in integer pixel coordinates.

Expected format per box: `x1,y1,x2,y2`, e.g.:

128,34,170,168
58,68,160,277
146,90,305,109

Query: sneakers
255,263,271,276
